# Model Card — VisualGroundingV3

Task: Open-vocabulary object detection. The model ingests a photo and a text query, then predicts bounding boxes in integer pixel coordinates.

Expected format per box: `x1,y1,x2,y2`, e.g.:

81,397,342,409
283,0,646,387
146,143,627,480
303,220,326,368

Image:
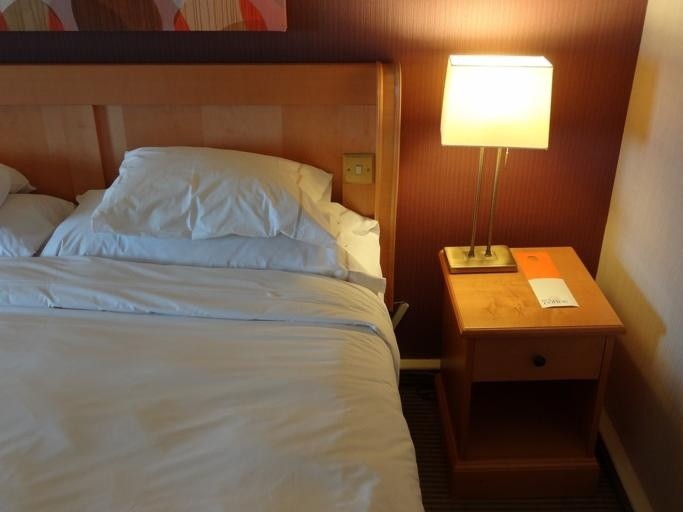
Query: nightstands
433,246,626,505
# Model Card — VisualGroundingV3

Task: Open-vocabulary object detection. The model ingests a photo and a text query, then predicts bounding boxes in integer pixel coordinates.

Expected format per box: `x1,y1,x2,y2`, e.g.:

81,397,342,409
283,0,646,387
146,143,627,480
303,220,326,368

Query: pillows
40,187,347,281
0,192,77,258
91,145,333,245
0,161,37,209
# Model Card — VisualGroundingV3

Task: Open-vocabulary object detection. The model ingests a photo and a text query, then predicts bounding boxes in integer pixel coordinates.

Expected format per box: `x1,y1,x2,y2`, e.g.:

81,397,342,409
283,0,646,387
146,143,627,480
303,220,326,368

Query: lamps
441,55,554,274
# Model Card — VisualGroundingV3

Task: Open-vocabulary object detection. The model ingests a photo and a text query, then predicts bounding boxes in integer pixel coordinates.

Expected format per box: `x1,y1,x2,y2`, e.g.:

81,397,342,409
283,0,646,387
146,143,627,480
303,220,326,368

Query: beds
0,61,426,512
